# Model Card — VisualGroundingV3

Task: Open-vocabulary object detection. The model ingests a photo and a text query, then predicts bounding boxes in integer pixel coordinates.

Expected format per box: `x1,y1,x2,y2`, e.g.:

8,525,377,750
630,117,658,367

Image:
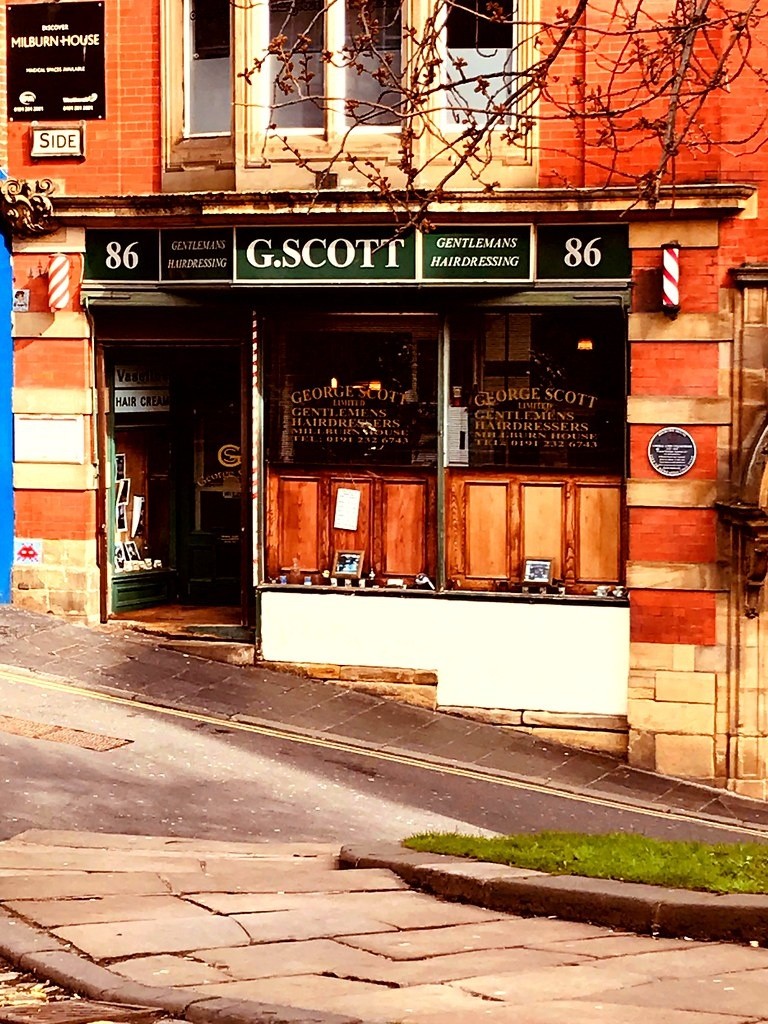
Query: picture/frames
520,556,555,587
331,549,366,579
